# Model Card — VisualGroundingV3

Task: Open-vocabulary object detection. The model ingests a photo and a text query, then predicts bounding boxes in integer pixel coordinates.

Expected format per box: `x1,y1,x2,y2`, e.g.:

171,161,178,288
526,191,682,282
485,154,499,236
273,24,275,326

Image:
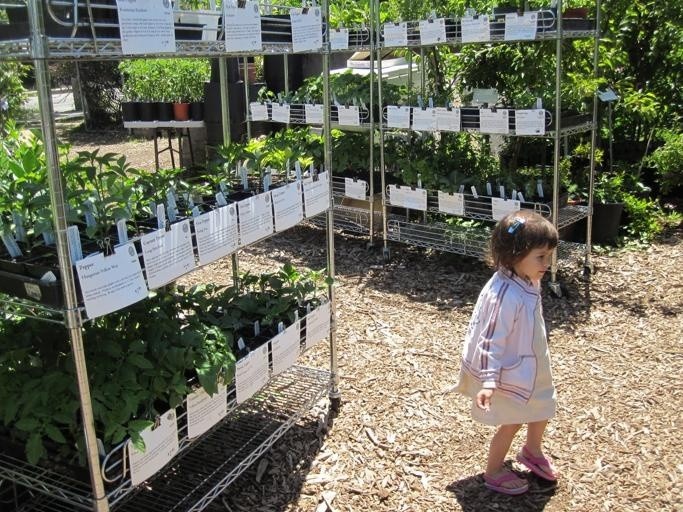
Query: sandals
516,447,555,481
483,469,530,496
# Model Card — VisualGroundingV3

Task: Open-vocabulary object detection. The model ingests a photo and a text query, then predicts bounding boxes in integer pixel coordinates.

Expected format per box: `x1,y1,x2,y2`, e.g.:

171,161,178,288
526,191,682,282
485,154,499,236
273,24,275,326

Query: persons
448,208,561,498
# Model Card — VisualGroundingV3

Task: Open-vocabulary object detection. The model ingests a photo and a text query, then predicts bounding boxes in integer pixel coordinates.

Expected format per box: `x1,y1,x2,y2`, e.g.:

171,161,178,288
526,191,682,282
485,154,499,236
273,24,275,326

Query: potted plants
565,40,655,239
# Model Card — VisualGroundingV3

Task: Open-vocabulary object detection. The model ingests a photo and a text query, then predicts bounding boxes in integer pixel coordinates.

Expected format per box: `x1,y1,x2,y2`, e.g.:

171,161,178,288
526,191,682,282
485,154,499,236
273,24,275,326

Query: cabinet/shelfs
383,0,603,296
1,2,341,510
236,6,412,252
